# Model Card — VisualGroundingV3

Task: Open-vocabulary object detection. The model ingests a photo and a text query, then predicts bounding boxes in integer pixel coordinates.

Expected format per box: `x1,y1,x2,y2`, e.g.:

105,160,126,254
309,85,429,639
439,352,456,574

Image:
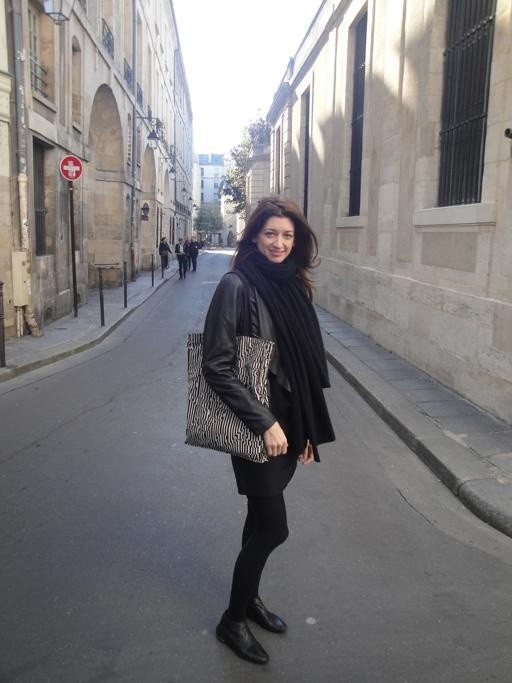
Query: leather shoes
215,609,270,665
245,596,287,634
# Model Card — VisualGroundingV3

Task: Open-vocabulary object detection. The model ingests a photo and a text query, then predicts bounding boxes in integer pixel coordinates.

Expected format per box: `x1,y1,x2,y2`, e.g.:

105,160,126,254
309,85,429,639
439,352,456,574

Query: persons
202,193,337,664
159,236,173,268
184,240,192,270
176,237,187,279
189,234,202,271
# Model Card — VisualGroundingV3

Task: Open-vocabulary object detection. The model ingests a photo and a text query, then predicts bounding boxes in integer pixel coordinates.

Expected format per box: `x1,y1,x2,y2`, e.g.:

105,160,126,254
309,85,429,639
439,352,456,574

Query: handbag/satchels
184,331,275,465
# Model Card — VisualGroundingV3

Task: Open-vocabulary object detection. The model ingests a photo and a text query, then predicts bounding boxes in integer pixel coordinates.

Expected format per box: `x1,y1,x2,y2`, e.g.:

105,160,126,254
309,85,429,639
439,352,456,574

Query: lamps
41,1,75,24
141,202,150,216
134,115,193,204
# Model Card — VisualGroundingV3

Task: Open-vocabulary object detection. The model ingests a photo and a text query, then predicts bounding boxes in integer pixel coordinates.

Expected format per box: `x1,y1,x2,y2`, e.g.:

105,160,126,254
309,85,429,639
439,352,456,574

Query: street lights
129,100,163,283
